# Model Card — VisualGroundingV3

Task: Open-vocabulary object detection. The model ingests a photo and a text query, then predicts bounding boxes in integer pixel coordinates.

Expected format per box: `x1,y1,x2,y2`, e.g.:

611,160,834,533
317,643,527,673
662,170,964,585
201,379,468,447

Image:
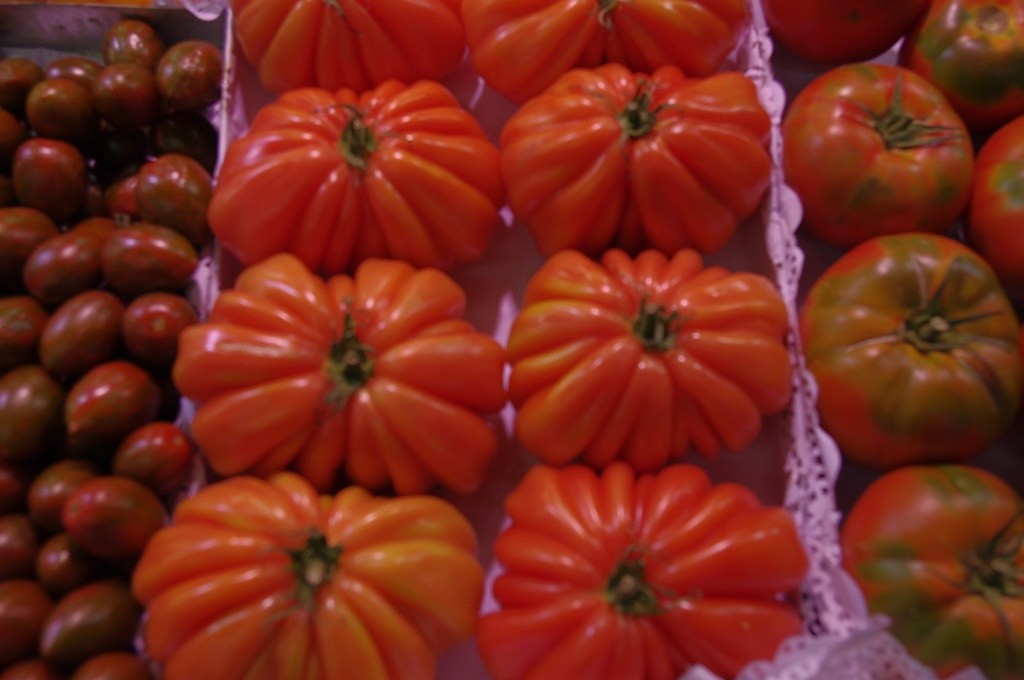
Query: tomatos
0,0,1024,680
